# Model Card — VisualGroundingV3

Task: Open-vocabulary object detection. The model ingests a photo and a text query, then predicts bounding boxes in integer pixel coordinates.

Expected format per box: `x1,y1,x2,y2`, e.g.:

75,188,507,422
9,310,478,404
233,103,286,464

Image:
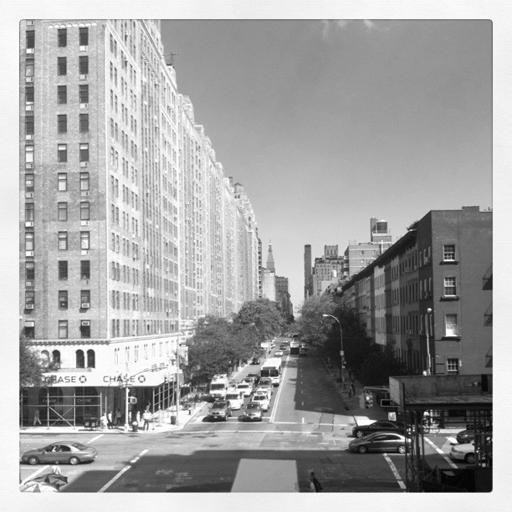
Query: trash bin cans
171,416,176,424
132,421,138,432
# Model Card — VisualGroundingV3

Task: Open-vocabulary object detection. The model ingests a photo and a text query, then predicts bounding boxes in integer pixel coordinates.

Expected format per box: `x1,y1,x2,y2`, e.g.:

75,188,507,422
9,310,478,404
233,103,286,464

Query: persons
307,478,316,491
33,408,43,425
100,402,152,432
309,471,322,491
52,459,61,489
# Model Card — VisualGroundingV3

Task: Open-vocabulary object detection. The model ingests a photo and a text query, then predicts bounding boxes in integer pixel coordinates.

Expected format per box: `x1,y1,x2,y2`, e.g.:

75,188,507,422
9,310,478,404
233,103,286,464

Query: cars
448,417,492,464
351,420,413,440
206,336,311,422
21,440,99,468
347,431,412,455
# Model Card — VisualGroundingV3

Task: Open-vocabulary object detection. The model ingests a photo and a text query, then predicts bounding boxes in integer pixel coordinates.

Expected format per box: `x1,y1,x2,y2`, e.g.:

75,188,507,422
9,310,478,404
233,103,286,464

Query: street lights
322,312,347,392
173,336,197,416
424,307,435,374
122,365,168,431
